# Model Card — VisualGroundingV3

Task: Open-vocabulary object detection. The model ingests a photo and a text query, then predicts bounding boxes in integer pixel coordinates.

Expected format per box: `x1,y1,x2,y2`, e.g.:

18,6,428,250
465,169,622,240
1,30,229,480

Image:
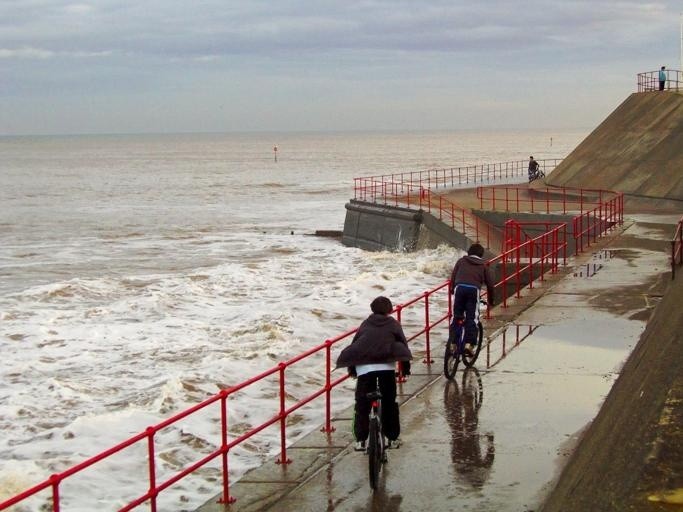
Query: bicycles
354,369,408,489
529,169,545,183
444,292,496,380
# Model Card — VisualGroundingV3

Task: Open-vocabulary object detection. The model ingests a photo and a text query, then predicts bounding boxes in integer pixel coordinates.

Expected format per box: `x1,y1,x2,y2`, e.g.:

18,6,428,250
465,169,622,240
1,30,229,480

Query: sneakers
353,441,366,451
451,342,474,358
388,435,403,449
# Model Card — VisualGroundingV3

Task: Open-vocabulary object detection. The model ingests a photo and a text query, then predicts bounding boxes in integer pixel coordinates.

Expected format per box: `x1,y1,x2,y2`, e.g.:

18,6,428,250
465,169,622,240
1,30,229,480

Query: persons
331,295,413,452
527,155,539,183
657,66,668,92
447,243,498,360
